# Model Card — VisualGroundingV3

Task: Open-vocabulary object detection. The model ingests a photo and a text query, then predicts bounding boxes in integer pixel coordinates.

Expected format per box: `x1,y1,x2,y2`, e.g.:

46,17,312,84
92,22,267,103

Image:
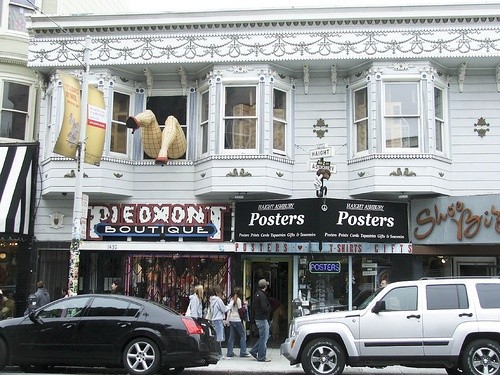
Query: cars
0,294,223,375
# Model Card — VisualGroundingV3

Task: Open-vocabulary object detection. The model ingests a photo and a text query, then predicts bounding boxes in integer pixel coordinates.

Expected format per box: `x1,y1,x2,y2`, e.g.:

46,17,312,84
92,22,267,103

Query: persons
185,285,203,317
299,289,312,316
0,279,128,322
249,279,271,362
380,280,391,287
226,288,252,357
126,110,187,166
209,285,233,356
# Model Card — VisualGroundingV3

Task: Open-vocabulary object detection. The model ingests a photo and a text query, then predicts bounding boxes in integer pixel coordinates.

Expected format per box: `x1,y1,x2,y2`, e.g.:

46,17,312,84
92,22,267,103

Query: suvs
280,276,500,375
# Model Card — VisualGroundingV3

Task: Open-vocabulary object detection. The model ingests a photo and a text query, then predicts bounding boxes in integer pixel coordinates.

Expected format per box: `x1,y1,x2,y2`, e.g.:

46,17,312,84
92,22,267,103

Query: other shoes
240,351,251,357
249,350,258,358
257,357,272,361
227,353,239,357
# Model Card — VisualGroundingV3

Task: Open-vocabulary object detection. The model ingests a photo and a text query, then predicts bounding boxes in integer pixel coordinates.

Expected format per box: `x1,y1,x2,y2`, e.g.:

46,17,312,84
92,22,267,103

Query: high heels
126,115,140,135
155,156,167,167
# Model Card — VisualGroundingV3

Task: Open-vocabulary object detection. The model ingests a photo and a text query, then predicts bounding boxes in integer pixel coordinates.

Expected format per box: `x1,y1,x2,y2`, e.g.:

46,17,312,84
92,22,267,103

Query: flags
0,145,40,238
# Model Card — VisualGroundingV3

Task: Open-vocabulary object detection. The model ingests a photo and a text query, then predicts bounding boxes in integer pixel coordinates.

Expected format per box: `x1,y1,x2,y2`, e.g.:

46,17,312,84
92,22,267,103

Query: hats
258,279,269,286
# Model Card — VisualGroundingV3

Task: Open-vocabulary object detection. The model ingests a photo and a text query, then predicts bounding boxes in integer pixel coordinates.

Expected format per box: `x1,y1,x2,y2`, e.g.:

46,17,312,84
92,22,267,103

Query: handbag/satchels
238,305,249,323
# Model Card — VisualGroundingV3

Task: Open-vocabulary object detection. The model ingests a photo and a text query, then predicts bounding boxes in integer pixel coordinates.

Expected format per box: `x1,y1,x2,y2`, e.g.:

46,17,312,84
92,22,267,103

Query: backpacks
203,297,221,320
